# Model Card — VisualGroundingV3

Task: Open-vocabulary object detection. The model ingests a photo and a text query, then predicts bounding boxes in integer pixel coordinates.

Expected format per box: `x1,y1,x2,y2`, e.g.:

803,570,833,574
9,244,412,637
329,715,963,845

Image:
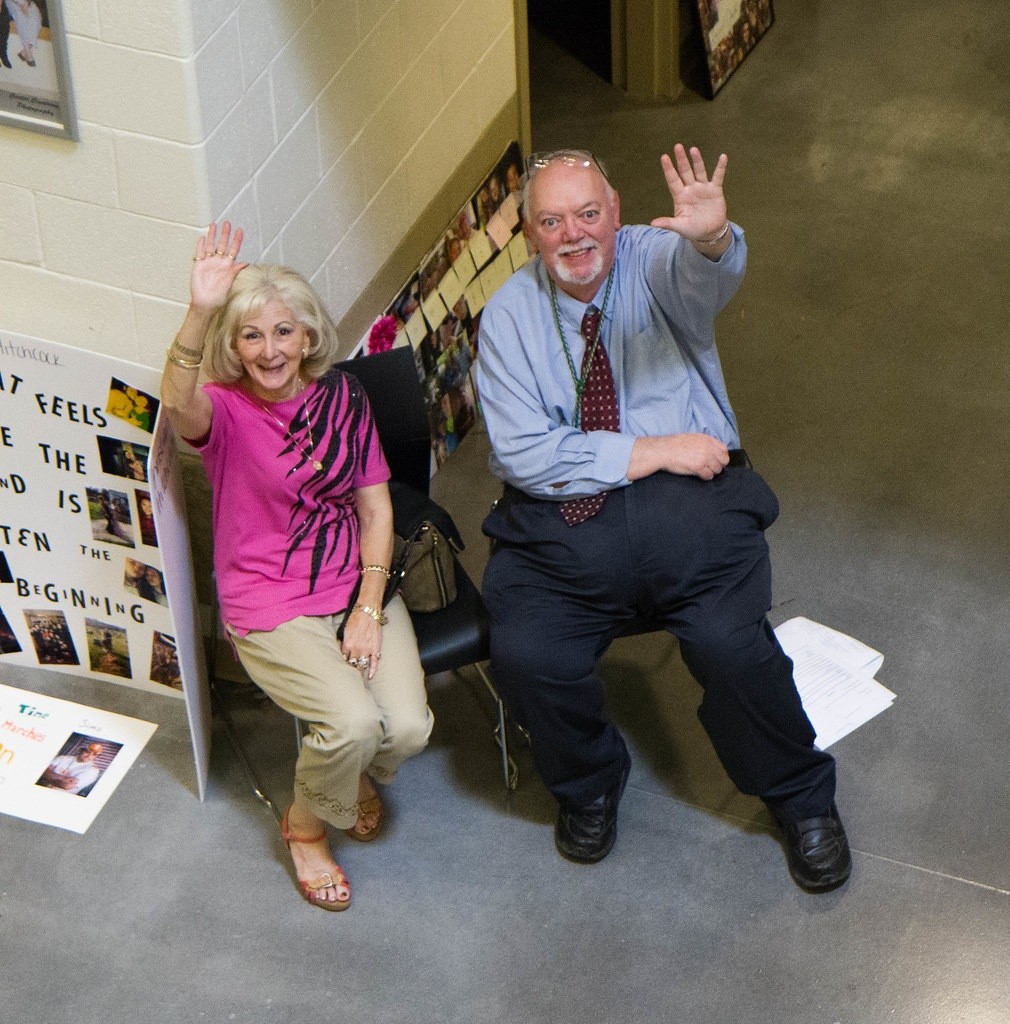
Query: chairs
209,344,532,824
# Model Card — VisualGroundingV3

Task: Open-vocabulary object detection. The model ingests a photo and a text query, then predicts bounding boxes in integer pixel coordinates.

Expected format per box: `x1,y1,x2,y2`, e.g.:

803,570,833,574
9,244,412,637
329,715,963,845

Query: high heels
282,802,351,912
345,791,382,842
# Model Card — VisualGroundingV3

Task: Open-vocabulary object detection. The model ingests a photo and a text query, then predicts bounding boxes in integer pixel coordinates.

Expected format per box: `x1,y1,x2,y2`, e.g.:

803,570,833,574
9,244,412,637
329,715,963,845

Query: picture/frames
0,0,79,142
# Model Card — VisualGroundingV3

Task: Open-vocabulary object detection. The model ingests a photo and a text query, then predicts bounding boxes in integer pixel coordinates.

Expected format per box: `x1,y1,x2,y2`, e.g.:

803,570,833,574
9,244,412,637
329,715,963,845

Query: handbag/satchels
335,520,457,641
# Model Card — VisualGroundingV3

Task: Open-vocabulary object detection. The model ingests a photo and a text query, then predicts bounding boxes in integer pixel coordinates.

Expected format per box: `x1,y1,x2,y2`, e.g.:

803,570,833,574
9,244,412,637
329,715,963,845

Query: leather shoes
554,752,631,862
773,798,852,893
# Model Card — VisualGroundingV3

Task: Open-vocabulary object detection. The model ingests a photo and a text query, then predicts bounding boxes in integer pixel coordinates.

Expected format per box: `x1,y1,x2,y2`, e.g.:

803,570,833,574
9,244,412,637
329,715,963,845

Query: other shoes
2,56,13,69
18,51,36,68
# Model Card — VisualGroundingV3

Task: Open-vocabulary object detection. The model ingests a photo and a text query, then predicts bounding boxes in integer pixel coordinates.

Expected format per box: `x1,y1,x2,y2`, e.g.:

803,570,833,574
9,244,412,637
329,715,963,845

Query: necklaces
255,374,322,470
548,260,616,430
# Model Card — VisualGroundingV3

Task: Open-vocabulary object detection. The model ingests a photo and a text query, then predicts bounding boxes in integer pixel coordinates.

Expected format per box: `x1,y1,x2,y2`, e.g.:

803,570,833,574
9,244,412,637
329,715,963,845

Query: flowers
368,314,398,355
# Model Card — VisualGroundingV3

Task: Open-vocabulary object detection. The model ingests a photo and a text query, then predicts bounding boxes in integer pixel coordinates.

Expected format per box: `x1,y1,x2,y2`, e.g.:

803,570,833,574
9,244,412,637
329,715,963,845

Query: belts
726,449,746,468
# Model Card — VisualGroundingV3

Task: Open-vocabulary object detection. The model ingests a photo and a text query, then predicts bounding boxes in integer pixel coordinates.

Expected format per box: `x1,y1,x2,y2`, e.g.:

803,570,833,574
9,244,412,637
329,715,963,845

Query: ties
559,307,621,527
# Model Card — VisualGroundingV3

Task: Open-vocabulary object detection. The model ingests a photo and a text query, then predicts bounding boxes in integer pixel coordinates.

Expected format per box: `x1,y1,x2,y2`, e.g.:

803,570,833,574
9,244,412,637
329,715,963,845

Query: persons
137,494,158,548
28,615,74,666
476,143,852,887
125,556,169,609
40,741,103,794
99,493,127,537
395,161,525,468
121,449,145,482
101,625,114,655
159,219,435,911
150,633,185,693
107,385,152,431
0,0,42,68
696,0,773,96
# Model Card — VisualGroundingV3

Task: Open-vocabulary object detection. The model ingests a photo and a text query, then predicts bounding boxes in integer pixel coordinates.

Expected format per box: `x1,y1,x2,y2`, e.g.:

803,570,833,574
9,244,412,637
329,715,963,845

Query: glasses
524,149,608,181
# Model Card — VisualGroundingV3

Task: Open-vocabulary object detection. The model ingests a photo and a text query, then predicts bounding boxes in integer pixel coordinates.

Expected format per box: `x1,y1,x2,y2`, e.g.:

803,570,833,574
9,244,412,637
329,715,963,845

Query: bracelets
692,219,729,245
360,565,391,579
167,338,206,371
352,601,389,625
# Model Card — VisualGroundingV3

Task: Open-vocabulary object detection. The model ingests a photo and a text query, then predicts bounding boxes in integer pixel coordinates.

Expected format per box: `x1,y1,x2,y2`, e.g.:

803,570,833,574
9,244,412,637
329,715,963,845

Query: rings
204,251,214,257
227,254,236,260
215,250,224,256
194,256,204,260
342,650,382,670
